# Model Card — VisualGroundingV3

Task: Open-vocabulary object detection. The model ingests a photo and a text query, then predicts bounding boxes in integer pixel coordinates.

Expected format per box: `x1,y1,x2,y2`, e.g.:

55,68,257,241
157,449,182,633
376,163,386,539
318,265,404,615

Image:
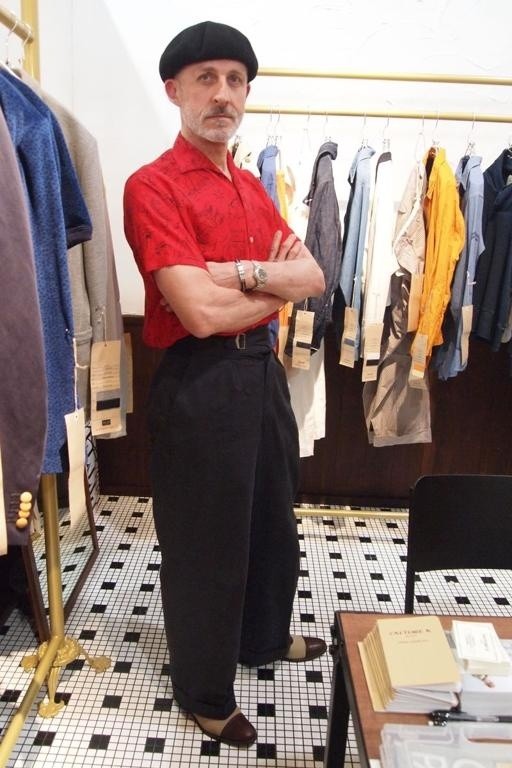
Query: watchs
248,259,269,293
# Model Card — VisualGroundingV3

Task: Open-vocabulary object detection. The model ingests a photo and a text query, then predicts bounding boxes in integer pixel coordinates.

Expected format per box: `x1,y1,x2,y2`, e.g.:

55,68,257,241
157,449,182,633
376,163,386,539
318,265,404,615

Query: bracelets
234,259,247,292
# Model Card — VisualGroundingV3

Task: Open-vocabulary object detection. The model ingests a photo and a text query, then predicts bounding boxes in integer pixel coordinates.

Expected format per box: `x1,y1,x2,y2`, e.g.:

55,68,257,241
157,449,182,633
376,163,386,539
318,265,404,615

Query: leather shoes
280,635,327,662
189,703,257,747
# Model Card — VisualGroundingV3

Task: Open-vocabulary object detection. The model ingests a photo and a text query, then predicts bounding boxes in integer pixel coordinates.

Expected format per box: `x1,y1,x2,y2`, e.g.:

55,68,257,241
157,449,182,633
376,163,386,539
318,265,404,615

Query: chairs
409,473,512,612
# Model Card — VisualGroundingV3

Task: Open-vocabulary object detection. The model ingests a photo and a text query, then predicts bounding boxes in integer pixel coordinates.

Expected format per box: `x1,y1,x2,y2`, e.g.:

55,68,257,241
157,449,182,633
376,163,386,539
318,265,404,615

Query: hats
159,21,258,82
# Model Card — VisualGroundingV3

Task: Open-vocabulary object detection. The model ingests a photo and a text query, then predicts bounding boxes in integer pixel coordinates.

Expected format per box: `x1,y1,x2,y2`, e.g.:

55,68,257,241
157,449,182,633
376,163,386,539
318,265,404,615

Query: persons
123,23,328,746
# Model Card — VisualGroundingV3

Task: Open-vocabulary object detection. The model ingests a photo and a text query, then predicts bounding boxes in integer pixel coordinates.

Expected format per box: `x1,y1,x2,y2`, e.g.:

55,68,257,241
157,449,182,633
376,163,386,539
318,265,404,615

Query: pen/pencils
431,710,500,722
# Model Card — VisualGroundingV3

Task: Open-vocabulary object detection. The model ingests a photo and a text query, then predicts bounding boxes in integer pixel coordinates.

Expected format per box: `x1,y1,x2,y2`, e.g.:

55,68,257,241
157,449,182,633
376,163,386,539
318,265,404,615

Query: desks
321,609,512,765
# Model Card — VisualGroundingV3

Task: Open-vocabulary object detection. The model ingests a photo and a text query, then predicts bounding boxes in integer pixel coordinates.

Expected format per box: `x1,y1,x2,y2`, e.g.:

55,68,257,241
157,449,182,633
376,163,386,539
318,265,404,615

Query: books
356,616,462,715
